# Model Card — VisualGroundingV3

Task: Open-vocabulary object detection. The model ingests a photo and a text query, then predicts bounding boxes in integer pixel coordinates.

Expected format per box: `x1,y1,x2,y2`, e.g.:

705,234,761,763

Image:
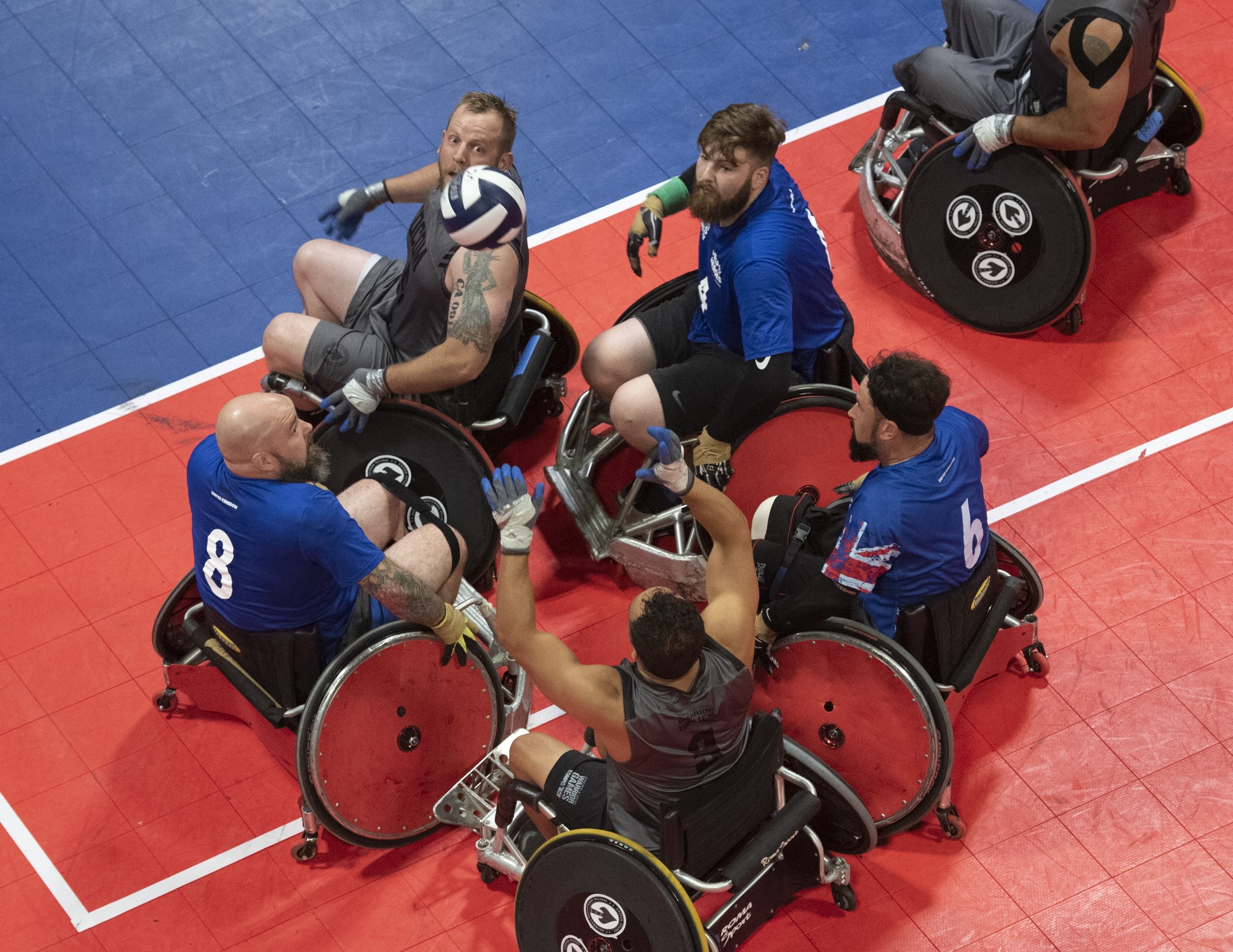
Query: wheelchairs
842,53,1206,342
256,289,583,592
738,482,1051,845
431,708,882,952
543,383,860,603
150,566,534,863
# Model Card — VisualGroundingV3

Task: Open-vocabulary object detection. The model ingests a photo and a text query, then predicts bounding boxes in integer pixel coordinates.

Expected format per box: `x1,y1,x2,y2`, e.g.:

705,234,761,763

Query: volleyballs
439,164,528,251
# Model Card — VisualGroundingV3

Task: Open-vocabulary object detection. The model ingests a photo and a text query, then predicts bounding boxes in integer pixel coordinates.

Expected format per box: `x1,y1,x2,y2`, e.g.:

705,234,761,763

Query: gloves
431,601,479,666
832,479,856,497
625,195,665,278
635,426,696,495
953,112,1013,172
691,426,734,493
317,179,388,242
320,368,396,436
750,610,779,676
482,464,545,554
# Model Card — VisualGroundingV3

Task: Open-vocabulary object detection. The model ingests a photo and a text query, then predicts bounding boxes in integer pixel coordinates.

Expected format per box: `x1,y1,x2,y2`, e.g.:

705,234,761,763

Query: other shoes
891,136,935,180
617,473,684,516
518,830,543,856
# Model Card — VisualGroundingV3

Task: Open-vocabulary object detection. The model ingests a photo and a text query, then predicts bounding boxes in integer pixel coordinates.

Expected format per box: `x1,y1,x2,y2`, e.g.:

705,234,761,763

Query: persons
752,349,987,674
892,0,1176,191
262,91,528,433
186,392,486,677
481,427,760,872
580,101,851,516
